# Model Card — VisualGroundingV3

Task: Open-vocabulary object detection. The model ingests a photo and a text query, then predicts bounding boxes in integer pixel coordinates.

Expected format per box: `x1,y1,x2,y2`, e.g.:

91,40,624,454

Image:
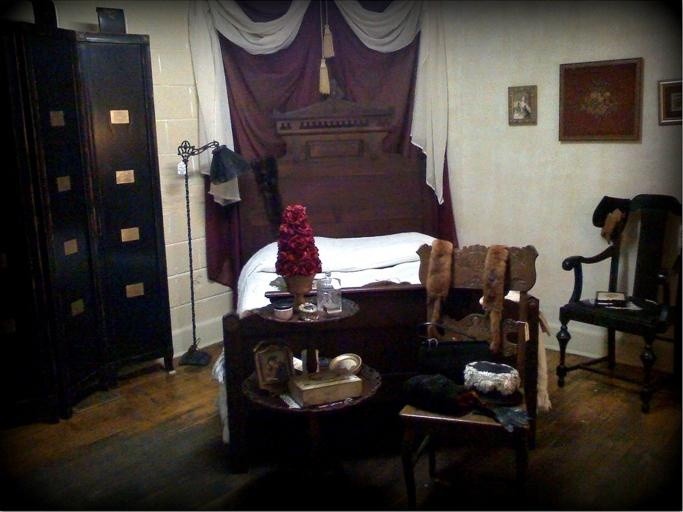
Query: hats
592,195,631,243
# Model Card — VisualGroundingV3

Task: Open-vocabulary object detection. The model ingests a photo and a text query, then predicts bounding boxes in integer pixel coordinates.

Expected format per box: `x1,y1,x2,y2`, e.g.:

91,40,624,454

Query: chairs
394,243,541,510
552,190,682,416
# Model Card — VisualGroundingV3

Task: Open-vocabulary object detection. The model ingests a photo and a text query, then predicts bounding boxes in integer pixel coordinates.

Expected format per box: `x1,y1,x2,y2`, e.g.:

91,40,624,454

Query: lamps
176,136,249,369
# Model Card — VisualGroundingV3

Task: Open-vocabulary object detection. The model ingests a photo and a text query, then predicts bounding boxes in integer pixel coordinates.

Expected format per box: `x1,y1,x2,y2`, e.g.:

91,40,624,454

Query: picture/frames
655,76,682,126
505,82,539,127
253,337,292,393
554,56,643,146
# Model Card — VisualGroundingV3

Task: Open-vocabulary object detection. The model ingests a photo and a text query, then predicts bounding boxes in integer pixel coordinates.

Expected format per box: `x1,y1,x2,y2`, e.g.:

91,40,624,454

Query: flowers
271,197,322,277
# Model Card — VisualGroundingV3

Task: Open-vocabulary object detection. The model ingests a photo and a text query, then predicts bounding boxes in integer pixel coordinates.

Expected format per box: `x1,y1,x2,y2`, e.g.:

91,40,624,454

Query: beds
206,94,552,478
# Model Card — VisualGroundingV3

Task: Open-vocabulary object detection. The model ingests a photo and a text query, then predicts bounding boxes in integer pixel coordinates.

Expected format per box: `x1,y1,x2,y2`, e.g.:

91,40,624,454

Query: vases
284,274,319,306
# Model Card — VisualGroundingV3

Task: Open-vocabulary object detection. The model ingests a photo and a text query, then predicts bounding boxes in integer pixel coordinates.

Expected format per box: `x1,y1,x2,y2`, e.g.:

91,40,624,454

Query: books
595,291,626,306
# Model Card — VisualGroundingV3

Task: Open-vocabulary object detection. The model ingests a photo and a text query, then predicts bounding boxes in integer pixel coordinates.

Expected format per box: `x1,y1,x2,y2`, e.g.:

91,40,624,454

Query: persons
514,96,532,120
266,356,286,381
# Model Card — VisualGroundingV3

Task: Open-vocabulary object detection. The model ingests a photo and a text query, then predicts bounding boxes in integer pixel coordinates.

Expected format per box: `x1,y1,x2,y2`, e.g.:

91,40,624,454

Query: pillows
260,231,437,275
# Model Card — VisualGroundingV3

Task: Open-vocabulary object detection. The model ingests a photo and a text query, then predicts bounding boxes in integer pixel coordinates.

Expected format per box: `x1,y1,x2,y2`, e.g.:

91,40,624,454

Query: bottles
315,270,343,314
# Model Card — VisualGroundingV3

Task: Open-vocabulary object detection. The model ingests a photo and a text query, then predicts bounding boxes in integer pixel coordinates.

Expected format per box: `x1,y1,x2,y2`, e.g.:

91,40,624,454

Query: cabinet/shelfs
75,30,172,381
0,23,104,425
241,292,388,510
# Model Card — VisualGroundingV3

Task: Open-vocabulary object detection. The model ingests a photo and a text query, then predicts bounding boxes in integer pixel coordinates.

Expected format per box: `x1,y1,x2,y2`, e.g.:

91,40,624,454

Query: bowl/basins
269,297,298,311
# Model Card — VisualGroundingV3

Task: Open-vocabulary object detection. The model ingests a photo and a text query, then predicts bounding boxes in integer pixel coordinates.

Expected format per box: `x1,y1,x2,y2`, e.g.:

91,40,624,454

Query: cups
298,303,316,322
274,307,293,322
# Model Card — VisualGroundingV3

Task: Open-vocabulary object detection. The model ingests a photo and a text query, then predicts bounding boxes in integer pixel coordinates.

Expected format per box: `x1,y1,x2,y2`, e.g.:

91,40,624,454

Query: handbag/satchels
420,339,493,386
